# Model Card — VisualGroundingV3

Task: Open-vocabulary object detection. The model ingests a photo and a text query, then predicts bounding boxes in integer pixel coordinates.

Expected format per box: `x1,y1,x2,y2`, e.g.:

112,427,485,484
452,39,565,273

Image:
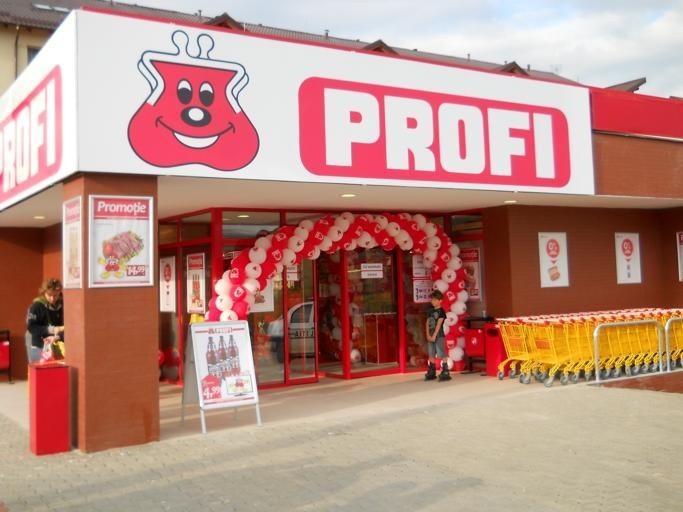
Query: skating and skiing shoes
424,369,452,381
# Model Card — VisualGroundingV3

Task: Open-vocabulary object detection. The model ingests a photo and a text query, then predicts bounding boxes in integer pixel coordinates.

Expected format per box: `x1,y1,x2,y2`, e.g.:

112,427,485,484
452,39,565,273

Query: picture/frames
459,247,482,302
88,195,154,288
159,256,176,312
676,231,683,282
186,254,206,315
62,196,83,290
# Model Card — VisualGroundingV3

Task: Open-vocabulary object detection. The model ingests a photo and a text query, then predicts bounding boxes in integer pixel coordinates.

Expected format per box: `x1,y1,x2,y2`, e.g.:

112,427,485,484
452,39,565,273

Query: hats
428,290,442,299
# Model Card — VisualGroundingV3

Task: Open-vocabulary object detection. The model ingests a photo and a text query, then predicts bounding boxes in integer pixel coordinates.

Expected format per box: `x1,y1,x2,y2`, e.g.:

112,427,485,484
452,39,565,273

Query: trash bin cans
484,323,513,377
29,364,70,456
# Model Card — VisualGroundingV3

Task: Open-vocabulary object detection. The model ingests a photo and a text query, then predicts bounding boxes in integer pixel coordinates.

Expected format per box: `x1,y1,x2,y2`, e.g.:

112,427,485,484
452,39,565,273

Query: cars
264,295,318,364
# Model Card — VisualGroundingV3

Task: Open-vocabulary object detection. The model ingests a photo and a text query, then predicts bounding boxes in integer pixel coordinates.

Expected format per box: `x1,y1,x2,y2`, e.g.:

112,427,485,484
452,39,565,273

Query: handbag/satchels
40,336,64,363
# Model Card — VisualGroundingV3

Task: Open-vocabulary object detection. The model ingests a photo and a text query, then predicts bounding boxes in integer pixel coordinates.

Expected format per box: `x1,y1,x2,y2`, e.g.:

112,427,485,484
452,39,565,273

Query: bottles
206,335,241,382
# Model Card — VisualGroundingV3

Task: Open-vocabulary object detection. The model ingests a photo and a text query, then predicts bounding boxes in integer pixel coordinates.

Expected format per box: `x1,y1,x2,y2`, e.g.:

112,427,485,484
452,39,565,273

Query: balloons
289,212,417,266
411,214,468,311
325,262,362,362
208,225,295,322
427,302,468,371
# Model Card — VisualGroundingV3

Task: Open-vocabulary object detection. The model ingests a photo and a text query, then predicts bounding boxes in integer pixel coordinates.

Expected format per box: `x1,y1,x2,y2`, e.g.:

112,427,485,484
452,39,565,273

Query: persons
425,290,449,377
27,279,63,363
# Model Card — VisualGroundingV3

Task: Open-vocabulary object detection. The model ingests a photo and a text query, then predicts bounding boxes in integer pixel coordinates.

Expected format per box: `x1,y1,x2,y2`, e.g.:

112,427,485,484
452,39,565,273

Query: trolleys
493,304,683,387
